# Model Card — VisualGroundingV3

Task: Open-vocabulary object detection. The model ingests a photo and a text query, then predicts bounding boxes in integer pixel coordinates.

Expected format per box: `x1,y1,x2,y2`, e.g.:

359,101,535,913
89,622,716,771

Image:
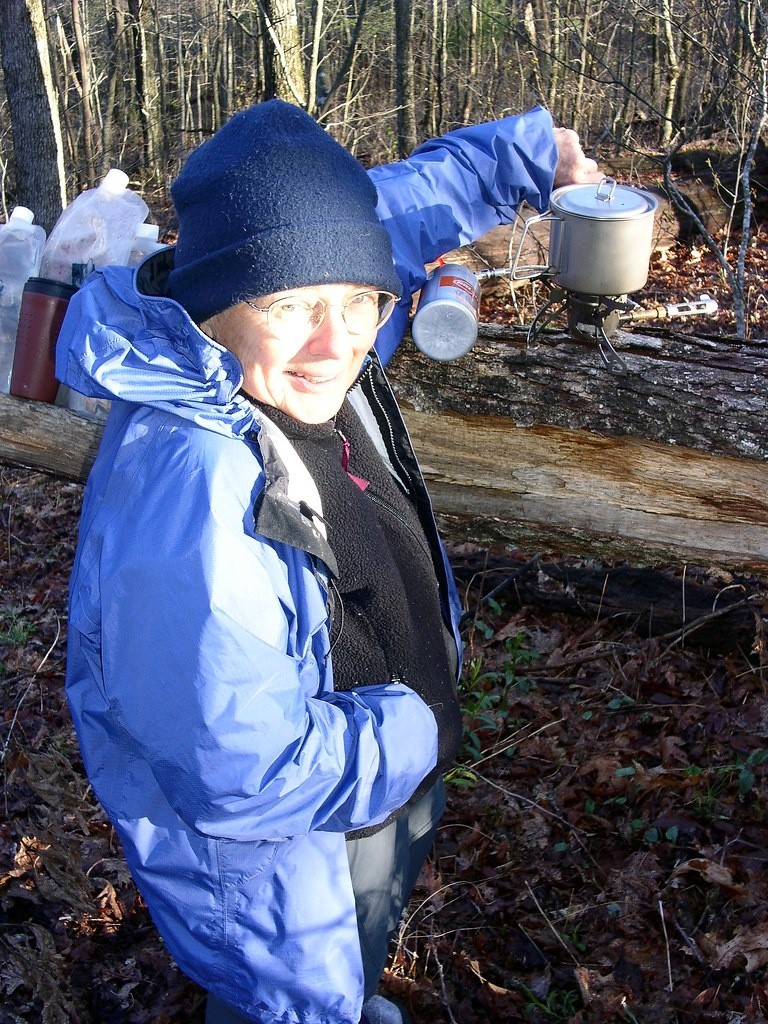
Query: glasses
244,277,402,337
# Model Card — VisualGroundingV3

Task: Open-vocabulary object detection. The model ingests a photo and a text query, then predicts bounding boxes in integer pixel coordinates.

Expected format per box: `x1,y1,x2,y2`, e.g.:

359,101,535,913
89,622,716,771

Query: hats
168,97,403,326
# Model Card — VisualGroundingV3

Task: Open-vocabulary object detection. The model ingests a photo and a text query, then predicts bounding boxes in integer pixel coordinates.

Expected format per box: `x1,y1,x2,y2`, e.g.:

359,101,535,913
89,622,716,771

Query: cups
9,277,80,403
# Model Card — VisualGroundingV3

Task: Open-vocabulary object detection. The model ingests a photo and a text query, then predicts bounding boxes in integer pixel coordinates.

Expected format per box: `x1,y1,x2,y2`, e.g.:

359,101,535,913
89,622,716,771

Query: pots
509,177,659,294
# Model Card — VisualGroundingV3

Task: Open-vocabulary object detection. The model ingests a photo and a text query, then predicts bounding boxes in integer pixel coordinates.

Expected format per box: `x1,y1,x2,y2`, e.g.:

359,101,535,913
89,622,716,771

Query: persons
57,98,608,1023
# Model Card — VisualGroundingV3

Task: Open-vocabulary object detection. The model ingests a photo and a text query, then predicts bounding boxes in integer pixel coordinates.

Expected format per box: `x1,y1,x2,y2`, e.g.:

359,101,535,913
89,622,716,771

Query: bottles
414,263,480,363
0,206,35,394
129,222,159,267
40,169,129,284
